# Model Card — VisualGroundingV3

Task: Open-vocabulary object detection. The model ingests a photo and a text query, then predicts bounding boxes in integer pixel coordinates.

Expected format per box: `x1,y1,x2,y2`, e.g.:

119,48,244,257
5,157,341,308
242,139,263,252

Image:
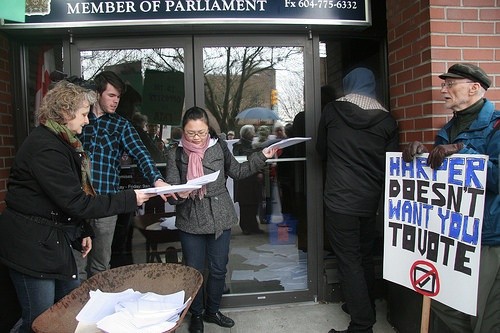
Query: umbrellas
235,108,279,120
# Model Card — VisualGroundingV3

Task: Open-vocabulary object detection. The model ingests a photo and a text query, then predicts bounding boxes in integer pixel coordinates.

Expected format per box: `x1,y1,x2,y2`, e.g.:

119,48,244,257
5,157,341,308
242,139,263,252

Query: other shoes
278,222,292,226
329,326,374,333
341,303,376,324
261,218,270,224
243,228,263,235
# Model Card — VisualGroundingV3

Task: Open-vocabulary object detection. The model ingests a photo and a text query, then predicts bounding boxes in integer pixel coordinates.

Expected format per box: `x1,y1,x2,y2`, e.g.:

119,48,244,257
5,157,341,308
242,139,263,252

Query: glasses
184,129,208,138
441,81,477,89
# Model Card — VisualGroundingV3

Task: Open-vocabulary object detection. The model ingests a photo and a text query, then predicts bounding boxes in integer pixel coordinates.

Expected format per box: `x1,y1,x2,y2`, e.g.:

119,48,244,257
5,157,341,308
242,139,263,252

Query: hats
438,64,491,91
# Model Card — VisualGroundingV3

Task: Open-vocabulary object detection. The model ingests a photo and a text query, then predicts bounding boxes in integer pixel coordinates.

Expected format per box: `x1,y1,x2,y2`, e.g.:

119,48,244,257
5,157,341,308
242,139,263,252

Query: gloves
427,142,463,169
403,140,426,161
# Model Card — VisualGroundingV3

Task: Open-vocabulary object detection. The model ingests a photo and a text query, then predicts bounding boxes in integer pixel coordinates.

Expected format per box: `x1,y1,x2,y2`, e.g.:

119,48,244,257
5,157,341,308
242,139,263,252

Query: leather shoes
203,309,234,328
189,313,204,333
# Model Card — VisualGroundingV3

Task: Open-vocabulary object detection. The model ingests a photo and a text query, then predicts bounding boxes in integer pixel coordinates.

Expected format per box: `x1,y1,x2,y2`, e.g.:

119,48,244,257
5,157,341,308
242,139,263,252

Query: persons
0,81,157,333
165,107,278,333
73,71,178,288
219,112,306,262
402,62,500,333
110,85,183,267
316,68,400,333
